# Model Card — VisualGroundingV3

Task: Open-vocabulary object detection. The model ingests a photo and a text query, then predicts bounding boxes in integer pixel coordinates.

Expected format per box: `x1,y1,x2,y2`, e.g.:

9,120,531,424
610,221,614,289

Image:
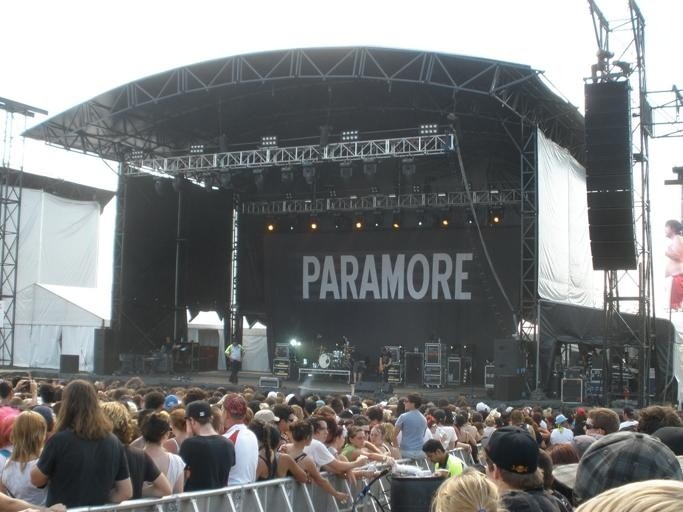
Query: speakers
561,378,583,404
258,376,282,389
354,381,393,394
494,339,522,402
585,81,639,270
94,329,118,374
60,355,78,373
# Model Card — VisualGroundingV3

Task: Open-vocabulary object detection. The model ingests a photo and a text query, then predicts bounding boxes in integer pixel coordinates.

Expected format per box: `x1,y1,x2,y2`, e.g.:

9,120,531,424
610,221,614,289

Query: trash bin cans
391,473,445,512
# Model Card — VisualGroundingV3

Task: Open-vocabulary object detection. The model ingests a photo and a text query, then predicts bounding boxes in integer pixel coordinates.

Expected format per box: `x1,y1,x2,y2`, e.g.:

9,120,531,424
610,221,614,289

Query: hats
554,414,569,425
164,395,178,412
224,394,247,417
476,402,490,413
253,409,280,425
572,426,683,499
184,400,211,419
485,425,540,475
550,427,575,445
259,391,295,410
576,408,586,417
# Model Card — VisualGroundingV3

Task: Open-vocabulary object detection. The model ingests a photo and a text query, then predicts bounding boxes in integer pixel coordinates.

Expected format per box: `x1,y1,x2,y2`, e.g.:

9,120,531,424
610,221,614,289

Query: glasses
586,423,599,430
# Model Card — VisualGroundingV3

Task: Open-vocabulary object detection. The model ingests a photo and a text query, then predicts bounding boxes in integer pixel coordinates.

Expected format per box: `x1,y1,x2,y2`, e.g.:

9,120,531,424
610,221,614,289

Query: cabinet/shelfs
258,339,449,389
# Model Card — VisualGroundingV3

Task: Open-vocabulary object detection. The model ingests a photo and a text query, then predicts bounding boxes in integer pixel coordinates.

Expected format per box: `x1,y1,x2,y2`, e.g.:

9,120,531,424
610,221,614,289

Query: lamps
128,149,144,156
415,124,439,136
151,157,419,200
340,128,361,141
189,143,205,156
260,133,278,148
259,205,506,234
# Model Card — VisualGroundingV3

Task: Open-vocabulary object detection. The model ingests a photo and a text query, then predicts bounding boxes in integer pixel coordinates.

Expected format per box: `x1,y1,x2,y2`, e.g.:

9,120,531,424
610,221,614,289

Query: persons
379,347,392,381
224,339,244,384
662,218,683,313
351,348,368,385
